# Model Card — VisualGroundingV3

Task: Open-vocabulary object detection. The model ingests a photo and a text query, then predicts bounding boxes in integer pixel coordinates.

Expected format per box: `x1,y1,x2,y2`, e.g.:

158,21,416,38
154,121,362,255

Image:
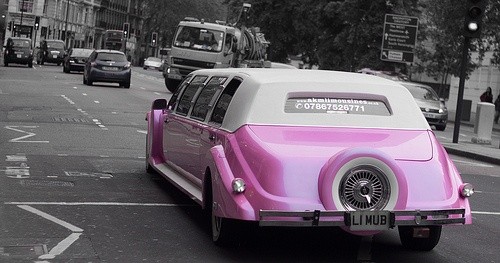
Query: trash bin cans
460,100,473,121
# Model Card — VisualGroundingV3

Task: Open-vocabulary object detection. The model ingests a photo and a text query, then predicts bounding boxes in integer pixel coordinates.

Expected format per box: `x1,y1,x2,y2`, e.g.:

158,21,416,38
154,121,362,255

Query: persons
493,94,500,125
479,87,494,103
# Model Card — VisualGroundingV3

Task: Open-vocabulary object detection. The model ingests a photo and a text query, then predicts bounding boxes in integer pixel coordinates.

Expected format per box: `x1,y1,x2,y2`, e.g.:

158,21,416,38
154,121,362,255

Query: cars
394,79,448,131
81,49,132,89
64,47,96,73
143,57,165,71
144,66,474,256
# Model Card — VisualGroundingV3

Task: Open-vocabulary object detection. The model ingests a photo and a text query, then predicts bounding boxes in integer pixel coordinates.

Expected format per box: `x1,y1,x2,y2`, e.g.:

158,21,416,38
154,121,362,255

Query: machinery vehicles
163,3,268,94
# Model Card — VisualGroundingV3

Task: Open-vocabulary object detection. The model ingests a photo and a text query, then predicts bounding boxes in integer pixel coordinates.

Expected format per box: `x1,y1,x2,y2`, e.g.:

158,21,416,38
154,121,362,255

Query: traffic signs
382,22,418,48
380,49,414,64
384,14,419,27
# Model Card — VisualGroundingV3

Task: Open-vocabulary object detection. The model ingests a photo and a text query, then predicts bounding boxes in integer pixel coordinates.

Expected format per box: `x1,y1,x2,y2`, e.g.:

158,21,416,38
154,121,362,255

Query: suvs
36,40,67,66
3,38,36,68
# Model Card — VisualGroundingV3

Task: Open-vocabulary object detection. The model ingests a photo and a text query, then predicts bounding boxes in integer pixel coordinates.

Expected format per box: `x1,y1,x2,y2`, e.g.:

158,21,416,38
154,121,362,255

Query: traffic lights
461,0,486,38
7,20,12,31
151,32,158,47
122,23,129,39
35,16,40,29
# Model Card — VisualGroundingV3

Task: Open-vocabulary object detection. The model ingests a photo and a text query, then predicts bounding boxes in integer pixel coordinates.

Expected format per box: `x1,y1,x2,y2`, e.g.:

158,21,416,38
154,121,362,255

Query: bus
103,30,127,53
